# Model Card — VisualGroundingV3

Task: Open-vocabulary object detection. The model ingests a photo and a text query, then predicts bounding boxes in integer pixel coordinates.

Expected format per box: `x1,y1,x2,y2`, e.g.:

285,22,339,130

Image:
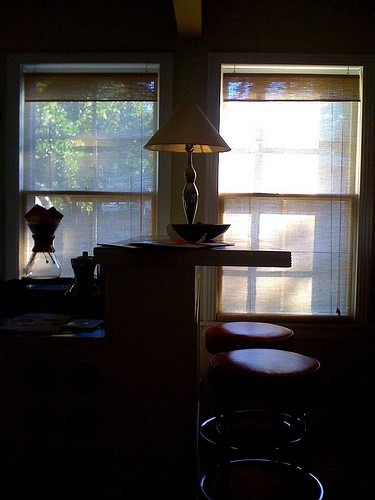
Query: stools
200,349,323,500
199,322,307,451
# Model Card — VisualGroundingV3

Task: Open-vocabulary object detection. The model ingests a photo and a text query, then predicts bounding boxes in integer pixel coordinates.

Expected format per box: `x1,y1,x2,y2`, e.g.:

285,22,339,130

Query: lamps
143,103,232,224
